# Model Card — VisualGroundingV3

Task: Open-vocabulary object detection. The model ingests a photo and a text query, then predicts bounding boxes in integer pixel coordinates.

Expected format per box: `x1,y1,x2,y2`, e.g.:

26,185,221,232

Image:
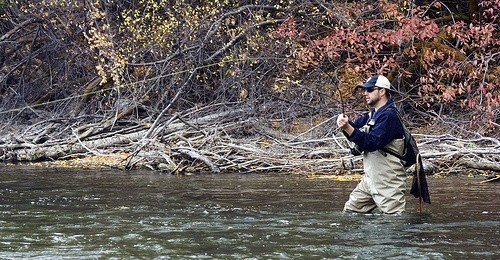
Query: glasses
361,88,381,92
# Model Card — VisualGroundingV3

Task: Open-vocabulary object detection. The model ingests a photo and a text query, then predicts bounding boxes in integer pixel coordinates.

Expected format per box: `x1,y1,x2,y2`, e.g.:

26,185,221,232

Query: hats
355,74,391,90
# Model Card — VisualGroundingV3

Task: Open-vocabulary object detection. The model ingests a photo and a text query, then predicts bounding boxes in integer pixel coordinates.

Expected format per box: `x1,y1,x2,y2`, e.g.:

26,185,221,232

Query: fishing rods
226,56,345,118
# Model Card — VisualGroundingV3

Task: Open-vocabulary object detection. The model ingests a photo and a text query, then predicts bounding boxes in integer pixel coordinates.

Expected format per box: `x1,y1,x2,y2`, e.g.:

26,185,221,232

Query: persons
337,74,407,216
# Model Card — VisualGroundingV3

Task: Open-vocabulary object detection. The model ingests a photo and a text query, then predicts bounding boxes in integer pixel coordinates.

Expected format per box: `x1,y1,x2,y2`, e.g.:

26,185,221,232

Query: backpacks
359,107,421,175
